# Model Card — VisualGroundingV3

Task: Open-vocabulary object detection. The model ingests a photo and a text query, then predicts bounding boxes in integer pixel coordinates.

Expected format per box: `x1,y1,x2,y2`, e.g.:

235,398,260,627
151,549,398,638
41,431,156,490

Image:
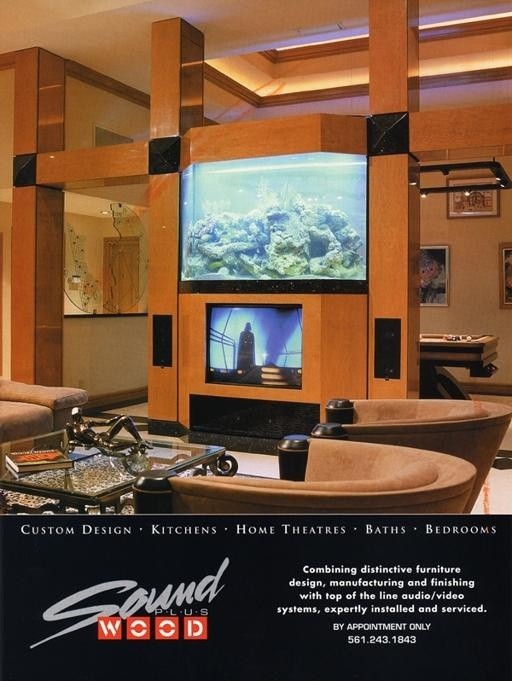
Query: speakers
153,315,172,367
374,318,400,379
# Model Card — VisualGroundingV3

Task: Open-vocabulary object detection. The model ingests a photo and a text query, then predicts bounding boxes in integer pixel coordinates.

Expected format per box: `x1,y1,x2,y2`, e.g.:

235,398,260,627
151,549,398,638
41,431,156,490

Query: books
5,463,34,479
5,449,75,473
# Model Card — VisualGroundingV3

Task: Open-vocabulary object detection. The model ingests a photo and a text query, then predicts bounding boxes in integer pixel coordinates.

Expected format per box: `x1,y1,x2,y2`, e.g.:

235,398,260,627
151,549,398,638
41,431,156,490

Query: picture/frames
420,245,449,310
496,241,512,311
445,176,500,221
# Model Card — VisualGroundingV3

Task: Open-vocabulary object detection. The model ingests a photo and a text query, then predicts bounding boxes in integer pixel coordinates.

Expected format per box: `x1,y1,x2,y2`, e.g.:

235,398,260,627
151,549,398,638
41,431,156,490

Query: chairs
130,397,512,514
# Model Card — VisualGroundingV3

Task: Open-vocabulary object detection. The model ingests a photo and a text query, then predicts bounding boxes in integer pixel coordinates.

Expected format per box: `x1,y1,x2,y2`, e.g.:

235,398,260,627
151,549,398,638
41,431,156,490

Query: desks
418,332,500,398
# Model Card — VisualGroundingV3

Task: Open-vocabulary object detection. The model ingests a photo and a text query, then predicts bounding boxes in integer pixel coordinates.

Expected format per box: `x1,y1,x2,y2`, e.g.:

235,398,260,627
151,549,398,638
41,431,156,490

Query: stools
0,380,88,442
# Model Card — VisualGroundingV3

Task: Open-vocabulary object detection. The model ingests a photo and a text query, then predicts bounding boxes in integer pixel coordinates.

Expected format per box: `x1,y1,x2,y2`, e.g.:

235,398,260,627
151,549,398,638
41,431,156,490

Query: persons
65,406,154,454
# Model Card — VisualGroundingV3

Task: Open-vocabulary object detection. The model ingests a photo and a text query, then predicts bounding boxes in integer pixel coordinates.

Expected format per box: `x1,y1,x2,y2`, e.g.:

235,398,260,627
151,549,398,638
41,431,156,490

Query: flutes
444,334,463,341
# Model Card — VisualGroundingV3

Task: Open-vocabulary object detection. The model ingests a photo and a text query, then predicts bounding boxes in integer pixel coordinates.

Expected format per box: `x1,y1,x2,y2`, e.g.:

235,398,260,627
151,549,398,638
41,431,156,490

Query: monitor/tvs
205,303,302,390
